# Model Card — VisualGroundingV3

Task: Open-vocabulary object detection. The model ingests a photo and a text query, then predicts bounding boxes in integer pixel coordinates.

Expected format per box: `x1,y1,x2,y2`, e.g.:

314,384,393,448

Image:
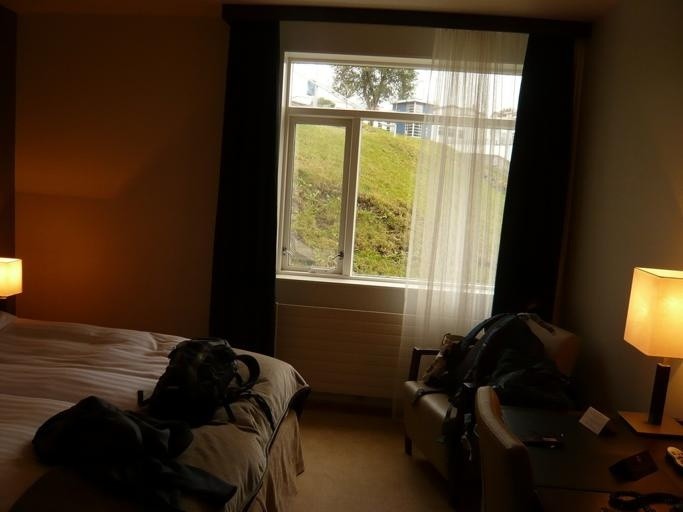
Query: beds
1,306,311,511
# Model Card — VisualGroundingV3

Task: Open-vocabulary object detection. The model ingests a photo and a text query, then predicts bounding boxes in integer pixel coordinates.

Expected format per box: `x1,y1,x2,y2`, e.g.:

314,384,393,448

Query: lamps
0,256,23,310
613,265,683,439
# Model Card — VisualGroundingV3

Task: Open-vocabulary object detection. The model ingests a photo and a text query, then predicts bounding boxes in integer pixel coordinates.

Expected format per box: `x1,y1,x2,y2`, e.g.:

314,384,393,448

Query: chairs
399,317,589,511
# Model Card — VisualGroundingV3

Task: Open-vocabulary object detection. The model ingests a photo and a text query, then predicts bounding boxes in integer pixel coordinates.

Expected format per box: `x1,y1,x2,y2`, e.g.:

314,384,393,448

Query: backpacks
137,335,260,421
422,311,579,414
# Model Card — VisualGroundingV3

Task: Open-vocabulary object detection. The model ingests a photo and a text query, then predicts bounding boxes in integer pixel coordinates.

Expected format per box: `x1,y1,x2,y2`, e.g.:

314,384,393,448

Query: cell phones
519,433,564,448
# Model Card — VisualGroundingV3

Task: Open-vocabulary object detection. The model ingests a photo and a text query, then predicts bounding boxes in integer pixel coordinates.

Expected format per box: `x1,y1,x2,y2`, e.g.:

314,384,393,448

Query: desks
500,403,682,512
